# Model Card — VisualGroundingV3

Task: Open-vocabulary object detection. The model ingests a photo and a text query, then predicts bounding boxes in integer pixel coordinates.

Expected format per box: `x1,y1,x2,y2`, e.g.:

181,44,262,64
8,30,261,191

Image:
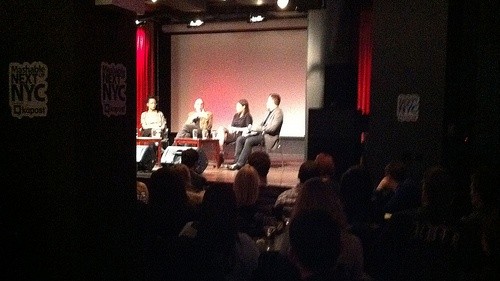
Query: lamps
247,5,266,23
187,11,205,28
134,15,150,27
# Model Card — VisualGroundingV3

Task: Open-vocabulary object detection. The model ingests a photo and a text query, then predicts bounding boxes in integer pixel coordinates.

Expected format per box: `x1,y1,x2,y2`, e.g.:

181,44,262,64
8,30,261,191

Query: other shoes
227,164,242,170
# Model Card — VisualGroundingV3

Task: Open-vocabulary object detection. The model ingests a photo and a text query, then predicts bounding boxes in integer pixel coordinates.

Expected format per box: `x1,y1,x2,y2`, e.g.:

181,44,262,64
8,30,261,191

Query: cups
212,129,217,139
202,130,208,140
193,129,197,140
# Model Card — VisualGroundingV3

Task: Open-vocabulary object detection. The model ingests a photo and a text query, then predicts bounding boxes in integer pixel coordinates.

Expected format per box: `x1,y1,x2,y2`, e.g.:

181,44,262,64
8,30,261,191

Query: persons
141,98,166,154
137,148,500,281
226,93,283,170
169,97,213,146
216,99,253,166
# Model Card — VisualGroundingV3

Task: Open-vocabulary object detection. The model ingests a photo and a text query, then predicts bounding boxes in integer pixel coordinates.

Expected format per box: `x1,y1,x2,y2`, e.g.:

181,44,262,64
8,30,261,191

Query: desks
174,136,220,169
135,137,162,165
